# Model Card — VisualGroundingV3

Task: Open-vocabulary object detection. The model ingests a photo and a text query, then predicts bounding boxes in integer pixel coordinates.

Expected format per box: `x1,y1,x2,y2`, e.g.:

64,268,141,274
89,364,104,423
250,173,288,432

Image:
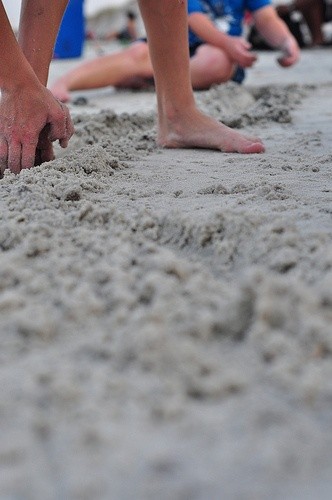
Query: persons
0,0,265,173
49,0,299,103
